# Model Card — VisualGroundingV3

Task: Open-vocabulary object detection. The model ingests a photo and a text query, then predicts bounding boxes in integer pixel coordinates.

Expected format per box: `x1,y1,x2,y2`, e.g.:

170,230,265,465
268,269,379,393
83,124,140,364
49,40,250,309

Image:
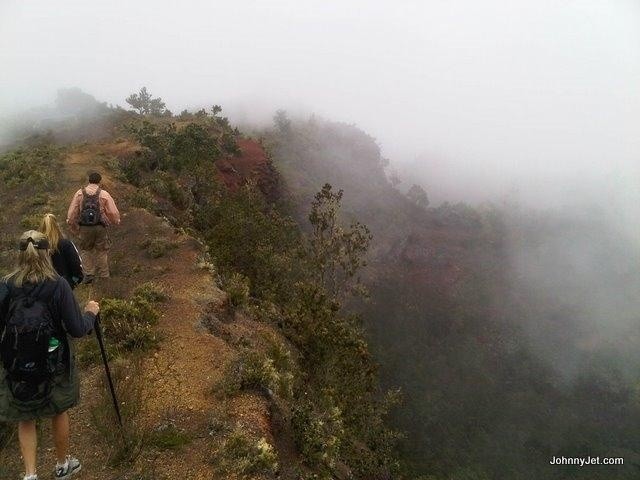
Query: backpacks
77,187,102,225
0,276,65,378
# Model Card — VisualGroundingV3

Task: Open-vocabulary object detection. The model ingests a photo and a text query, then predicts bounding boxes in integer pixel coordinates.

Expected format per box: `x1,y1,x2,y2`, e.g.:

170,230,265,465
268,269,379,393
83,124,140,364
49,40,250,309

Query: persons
64,172,120,283
35,211,81,293
0,229,100,480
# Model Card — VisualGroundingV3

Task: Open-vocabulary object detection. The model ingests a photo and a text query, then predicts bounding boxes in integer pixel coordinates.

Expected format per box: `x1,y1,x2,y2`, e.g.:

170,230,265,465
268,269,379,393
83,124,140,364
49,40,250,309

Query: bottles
48,337,63,370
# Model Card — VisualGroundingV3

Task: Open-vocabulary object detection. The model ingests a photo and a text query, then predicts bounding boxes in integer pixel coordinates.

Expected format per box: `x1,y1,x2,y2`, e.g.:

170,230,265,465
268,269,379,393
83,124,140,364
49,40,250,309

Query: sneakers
23,473,38,480
54,455,81,480
